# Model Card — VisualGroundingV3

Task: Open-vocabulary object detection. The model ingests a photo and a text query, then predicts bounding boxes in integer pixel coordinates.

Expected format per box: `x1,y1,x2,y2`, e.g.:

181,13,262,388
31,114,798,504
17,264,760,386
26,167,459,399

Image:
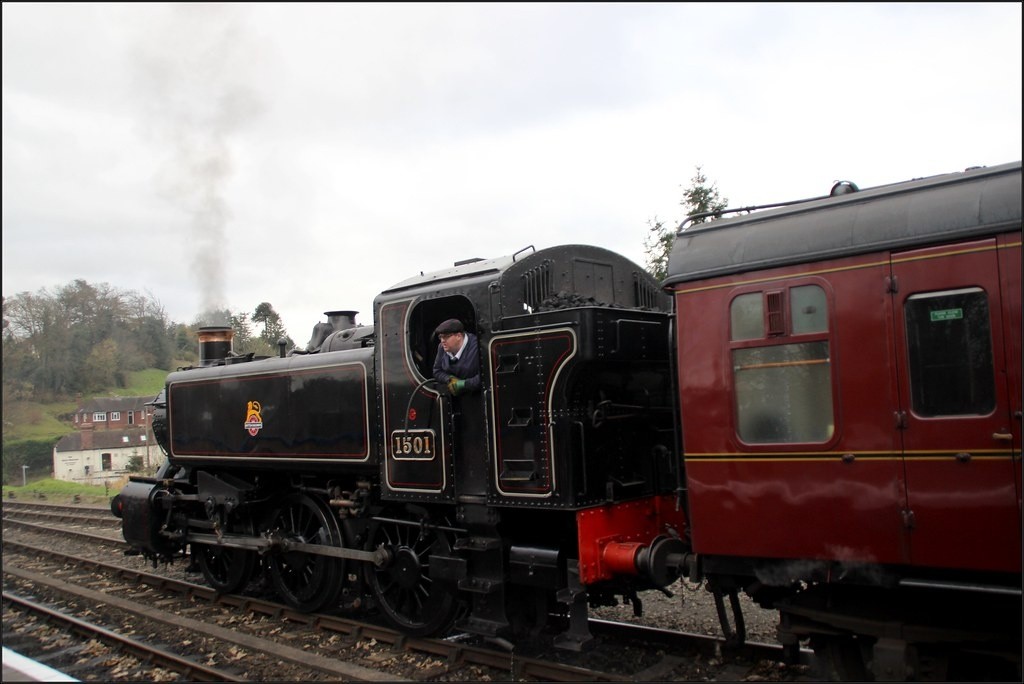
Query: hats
436,319,465,334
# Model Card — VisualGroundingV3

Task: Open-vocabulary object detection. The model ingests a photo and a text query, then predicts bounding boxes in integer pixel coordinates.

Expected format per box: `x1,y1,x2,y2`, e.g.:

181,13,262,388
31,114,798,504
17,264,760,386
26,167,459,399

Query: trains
110,159,1024,684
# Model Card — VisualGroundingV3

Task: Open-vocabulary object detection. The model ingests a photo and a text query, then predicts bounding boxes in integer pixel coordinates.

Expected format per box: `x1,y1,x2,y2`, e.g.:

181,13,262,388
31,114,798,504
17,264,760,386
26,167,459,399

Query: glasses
438,333,455,339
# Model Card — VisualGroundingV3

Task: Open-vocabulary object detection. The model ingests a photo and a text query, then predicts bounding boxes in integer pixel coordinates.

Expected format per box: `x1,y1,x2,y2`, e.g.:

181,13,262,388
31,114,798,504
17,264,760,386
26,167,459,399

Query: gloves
447,375,465,396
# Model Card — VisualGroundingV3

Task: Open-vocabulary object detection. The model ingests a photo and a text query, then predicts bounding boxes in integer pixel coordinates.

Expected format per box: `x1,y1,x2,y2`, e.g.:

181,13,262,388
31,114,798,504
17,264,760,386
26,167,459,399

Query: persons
432,318,488,495
